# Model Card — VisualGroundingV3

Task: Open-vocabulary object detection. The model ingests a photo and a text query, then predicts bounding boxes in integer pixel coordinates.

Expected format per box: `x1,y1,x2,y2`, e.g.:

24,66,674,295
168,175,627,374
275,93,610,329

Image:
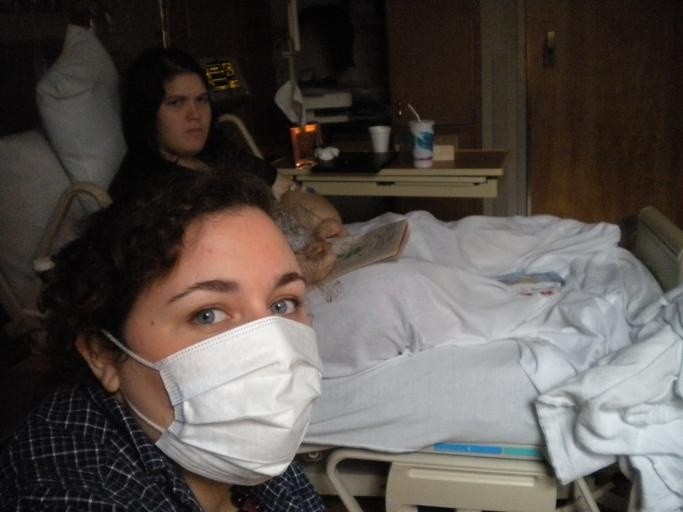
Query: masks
99,316,323,487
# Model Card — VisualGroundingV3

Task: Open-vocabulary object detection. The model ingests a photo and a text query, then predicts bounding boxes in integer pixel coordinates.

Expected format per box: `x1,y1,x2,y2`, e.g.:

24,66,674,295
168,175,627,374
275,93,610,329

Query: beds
0,113,683,512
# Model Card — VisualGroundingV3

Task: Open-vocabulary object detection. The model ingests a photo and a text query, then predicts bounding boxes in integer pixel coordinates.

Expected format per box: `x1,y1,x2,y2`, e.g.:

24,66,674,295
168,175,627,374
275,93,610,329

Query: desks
272,147,512,225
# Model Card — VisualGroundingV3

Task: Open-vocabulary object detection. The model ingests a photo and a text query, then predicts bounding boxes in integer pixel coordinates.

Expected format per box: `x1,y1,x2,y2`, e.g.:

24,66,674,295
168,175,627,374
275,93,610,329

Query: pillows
31,21,131,214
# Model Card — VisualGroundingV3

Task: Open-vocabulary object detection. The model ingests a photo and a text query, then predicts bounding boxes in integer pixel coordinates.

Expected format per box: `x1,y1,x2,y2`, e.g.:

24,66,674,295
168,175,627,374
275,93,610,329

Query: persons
0,168,329,512
108,47,343,286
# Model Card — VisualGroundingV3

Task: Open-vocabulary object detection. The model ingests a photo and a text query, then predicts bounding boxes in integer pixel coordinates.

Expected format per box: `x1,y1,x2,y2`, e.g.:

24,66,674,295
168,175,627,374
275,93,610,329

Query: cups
409,120,435,169
368,126,392,152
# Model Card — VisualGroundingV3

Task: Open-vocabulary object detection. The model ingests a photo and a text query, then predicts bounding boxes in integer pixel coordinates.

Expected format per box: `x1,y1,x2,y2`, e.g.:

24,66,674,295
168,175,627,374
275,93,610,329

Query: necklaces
230,485,263,512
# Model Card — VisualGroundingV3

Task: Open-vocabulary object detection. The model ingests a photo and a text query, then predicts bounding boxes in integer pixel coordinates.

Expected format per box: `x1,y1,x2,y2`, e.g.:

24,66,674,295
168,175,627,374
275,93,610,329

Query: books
317,218,410,286
